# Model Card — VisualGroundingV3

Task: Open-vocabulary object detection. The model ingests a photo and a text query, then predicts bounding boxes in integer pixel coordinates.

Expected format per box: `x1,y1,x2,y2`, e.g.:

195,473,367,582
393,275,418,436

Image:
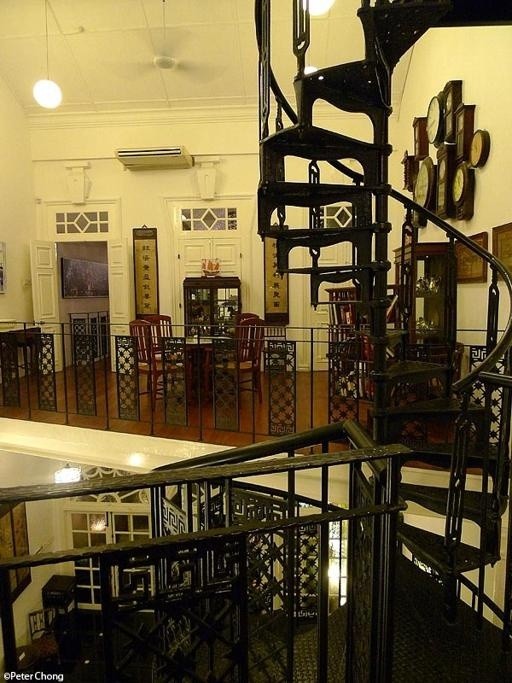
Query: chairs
128,313,265,414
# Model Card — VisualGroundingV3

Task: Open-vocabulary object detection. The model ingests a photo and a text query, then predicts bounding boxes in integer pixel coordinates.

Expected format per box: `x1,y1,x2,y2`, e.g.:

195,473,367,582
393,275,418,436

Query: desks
1,326,41,385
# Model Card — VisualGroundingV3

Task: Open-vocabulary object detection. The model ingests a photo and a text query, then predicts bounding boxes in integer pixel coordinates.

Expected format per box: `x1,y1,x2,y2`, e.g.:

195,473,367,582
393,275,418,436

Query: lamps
32,1,65,112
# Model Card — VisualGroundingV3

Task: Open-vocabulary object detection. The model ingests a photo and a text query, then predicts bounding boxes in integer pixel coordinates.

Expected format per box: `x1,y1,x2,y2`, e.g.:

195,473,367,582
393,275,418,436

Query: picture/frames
453,223,511,283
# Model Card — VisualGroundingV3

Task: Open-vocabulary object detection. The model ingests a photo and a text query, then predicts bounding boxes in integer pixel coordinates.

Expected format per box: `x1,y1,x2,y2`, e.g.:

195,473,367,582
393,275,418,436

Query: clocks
400,80,491,230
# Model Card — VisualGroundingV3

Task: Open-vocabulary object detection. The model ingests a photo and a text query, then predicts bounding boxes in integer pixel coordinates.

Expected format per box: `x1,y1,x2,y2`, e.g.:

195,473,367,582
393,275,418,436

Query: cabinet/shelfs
182,277,241,349
40,574,82,634
393,242,457,366
